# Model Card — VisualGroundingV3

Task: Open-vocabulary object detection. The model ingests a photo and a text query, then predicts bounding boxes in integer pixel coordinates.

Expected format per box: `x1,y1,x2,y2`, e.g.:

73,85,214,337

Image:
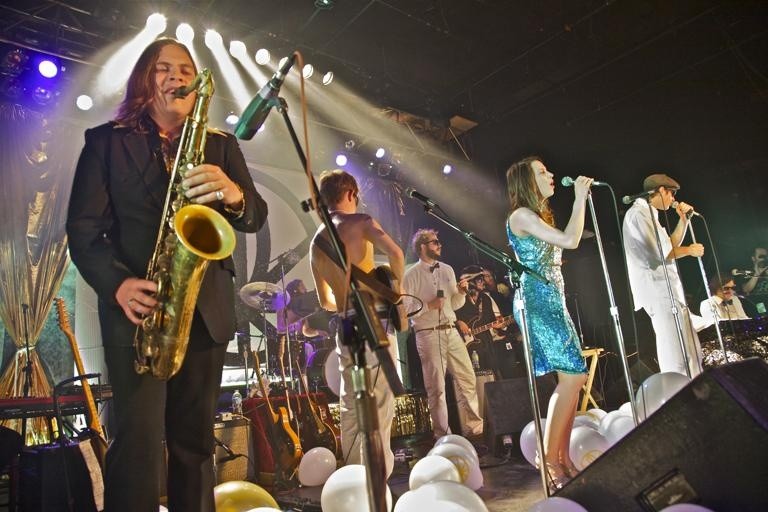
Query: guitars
277,337,309,454
289,339,342,459
374,265,408,332
458,315,514,348
251,351,304,474
53,297,108,443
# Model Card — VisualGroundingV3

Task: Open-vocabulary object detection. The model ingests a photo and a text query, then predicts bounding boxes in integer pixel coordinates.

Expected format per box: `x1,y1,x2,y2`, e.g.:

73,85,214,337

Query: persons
277,279,314,373
308,170,404,480
453,266,504,417
742,247,768,293
506,155,594,495
65,37,267,512
483,269,522,380
622,175,704,379
403,229,483,441
699,272,752,325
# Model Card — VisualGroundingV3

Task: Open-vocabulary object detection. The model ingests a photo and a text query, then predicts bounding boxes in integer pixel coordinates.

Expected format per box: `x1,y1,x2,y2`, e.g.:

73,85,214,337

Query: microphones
729,265,755,278
437,289,446,324
232,55,297,141
404,185,435,208
622,189,658,204
672,200,700,219
561,176,605,186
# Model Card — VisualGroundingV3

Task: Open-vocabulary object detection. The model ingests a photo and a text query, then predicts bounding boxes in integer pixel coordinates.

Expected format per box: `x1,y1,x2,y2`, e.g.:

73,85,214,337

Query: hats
644,174,680,190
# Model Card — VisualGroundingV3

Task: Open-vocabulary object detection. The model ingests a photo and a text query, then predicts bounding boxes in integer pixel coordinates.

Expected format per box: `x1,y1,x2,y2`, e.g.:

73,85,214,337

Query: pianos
698,316,768,344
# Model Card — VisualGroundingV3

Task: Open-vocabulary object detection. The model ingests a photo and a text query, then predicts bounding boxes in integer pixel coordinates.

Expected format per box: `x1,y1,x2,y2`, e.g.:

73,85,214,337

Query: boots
467,433,508,468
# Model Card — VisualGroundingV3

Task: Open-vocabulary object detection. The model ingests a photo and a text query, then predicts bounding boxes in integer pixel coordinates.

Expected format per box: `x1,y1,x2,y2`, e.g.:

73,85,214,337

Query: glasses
427,240,439,245
723,287,736,290
671,190,677,196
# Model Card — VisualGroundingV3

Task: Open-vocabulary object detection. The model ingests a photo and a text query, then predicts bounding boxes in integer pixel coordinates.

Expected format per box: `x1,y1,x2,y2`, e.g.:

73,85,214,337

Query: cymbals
240,282,291,311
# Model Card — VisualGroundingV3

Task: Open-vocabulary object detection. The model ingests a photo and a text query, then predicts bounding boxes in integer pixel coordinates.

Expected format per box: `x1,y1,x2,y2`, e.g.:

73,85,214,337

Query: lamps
290,54,313,80
270,45,290,72
0,42,70,114
308,53,333,87
246,39,270,65
361,137,387,162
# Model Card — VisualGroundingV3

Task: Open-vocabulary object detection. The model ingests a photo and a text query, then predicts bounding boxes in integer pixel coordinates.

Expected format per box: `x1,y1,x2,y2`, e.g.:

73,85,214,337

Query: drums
304,346,341,404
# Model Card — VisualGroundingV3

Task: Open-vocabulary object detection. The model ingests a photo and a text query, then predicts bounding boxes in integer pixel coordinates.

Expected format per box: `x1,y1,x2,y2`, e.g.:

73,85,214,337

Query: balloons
247,507,281,512
658,503,713,512
296,433,489,512
528,497,588,512
212,481,280,512
519,373,691,471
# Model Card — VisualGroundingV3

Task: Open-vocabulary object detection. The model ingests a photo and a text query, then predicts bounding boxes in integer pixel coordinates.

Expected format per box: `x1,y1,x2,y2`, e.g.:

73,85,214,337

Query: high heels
534,452,580,492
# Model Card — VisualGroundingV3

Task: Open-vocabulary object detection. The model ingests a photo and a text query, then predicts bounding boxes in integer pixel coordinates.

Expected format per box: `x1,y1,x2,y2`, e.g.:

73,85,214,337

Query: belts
416,325,456,333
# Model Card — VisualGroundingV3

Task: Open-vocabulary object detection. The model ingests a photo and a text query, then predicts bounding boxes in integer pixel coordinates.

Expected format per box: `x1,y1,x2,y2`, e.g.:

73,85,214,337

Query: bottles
472,351,480,372
232,390,243,420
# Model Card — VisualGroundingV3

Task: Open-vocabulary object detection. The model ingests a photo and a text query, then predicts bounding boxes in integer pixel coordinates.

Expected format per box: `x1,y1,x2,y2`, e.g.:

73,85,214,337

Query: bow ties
722,299,732,305
430,263,439,273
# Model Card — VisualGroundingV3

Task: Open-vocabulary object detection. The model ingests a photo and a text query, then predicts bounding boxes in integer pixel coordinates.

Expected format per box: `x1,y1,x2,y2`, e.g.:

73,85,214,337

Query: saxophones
133,68,236,378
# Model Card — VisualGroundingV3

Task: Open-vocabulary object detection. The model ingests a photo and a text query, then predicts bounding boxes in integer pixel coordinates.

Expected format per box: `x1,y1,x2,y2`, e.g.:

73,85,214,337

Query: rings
216,191,224,201
128,299,135,308
